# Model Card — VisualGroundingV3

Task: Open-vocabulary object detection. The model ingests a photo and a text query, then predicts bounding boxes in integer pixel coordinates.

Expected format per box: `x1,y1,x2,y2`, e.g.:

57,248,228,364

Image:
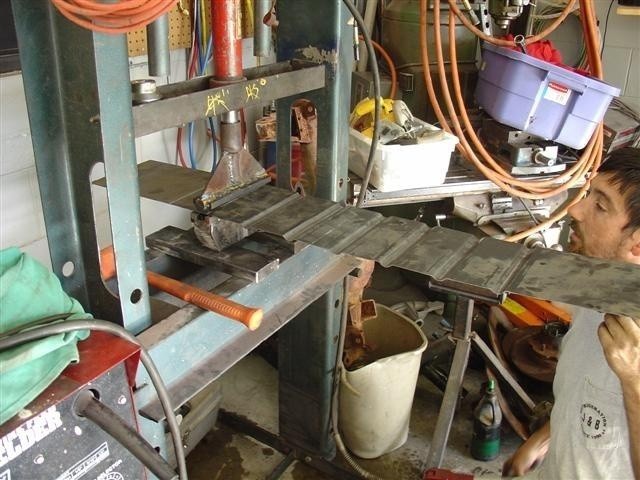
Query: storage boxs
347,114,460,193
474,41,622,151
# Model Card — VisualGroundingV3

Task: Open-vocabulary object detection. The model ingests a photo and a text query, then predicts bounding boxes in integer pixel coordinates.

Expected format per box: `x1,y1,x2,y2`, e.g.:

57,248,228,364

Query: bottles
472,380,503,462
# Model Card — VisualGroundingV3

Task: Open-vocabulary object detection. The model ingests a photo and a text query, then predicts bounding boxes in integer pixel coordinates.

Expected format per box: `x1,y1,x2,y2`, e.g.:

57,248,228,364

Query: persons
503,147,640,480
